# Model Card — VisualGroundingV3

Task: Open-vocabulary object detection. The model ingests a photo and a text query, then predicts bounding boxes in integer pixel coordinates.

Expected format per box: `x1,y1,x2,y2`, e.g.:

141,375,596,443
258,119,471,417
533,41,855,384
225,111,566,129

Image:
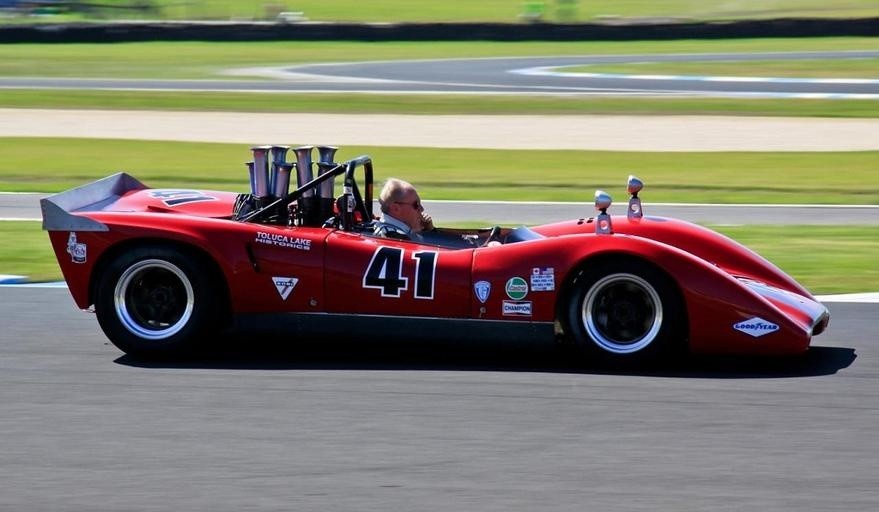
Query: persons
369,176,506,249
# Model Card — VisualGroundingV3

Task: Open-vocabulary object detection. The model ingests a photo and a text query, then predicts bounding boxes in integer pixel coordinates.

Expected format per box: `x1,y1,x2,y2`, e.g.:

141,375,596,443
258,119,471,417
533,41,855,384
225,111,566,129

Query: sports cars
39,143,833,365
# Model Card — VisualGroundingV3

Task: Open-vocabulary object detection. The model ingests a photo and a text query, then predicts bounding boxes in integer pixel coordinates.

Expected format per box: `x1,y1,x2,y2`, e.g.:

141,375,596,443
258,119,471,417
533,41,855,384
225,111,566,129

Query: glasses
392,200,419,209
